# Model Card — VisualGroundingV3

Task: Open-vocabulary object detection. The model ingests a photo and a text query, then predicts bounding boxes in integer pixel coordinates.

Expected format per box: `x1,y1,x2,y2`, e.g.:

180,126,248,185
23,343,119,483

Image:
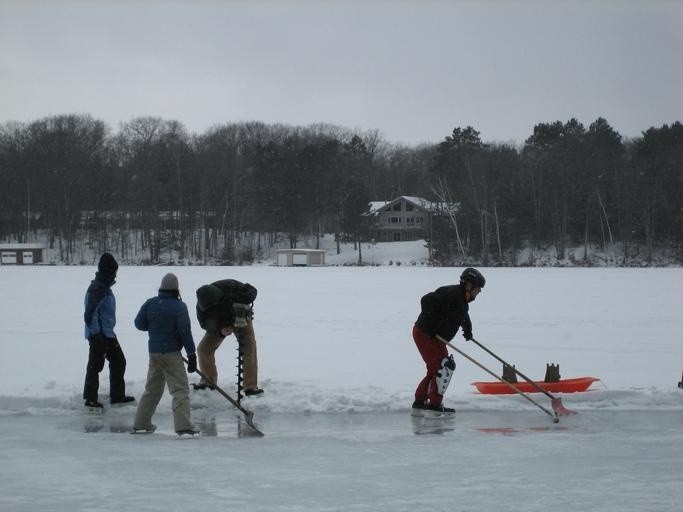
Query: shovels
183,356,266,436
470,336,577,415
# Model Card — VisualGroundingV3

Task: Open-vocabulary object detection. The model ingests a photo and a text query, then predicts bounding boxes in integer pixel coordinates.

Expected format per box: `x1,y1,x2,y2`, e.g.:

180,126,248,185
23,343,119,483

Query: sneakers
177,426,200,434
425,403,456,413
412,399,427,411
193,383,214,389
110,396,135,404
244,388,263,396
132,425,157,431
83,400,106,408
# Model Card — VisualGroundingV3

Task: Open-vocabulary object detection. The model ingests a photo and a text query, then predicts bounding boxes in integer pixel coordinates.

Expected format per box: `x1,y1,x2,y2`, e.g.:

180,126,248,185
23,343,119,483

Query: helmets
459,268,485,289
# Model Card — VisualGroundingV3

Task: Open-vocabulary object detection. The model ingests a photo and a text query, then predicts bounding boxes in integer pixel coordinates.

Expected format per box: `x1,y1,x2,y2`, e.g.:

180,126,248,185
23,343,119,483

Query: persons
192,279,265,397
130,273,202,435
411,266,486,415
83,250,135,409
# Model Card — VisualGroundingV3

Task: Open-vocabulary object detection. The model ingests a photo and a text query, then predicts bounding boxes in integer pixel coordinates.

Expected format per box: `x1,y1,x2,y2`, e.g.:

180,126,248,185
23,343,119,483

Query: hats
159,272,180,290
98,253,120,273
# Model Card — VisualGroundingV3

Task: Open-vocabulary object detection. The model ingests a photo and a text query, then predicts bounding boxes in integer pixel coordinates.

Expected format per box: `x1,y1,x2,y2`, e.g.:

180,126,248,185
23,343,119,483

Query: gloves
94,333,116,352
461,330,473,340
428,328,445,341
187,353,197,373
